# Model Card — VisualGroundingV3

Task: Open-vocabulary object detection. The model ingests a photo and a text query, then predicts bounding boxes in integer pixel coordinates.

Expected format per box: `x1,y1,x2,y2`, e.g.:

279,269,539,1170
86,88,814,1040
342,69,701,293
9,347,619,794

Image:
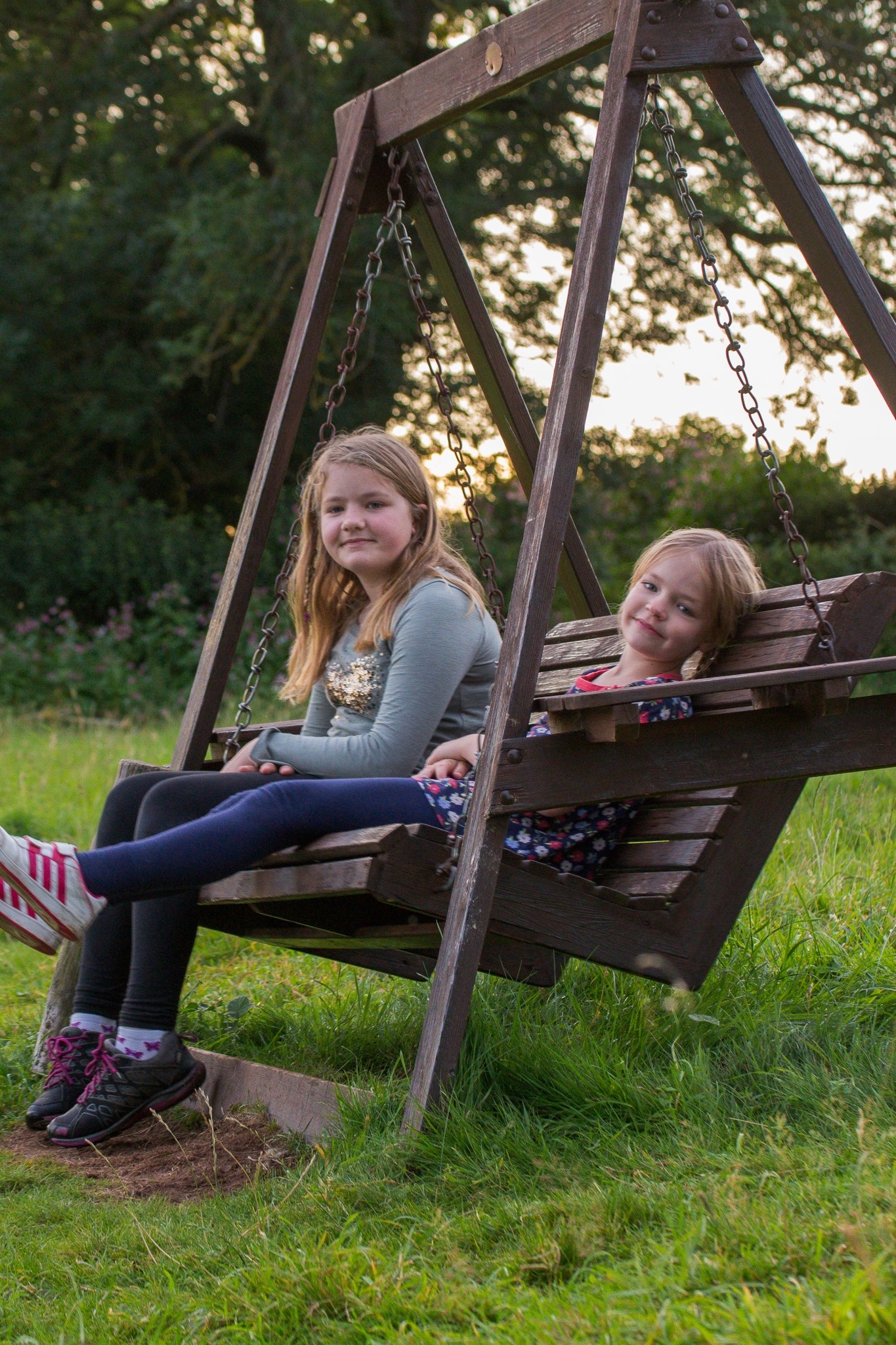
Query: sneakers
0,827,108,942
47,1031,206,1146
0,875,64,955
25,1026,115,1132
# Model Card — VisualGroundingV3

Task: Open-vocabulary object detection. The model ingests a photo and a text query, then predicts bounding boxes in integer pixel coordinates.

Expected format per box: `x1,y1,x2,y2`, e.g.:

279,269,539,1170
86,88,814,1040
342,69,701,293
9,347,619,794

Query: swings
197,73,895,998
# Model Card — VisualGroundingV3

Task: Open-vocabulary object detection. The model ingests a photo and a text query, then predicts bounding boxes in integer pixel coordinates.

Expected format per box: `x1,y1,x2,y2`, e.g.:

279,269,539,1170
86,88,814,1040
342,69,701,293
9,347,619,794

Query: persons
25,432,502,1147
0,527,763,955
